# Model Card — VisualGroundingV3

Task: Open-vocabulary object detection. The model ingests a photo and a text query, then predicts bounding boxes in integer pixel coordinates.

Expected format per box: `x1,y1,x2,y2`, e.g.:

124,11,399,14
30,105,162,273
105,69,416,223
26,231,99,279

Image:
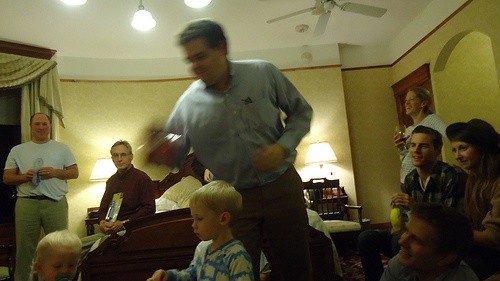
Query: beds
82,153,343,281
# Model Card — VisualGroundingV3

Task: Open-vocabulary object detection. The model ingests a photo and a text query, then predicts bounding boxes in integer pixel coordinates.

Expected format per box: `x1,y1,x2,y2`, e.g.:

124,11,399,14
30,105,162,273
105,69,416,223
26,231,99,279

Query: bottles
390,205,402,233
105,201,116,222
32,156,43,186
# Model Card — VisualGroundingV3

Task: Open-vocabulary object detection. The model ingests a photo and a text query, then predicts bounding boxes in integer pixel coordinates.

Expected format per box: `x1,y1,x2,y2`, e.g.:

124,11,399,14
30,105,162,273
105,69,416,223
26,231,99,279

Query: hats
445,119,500,154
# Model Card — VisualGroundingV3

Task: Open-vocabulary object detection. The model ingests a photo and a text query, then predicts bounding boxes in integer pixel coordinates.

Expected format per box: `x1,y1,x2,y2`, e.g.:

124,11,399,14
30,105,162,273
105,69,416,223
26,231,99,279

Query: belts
30,196,49,200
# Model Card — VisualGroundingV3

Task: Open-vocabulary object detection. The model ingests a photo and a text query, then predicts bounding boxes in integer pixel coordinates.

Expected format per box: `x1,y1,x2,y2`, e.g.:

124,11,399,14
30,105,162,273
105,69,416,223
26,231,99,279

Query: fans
266,0,388,37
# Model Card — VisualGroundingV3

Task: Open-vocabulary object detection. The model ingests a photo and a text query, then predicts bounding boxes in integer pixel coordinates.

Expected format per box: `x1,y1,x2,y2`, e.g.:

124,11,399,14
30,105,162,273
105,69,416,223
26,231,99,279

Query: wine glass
393,127,410,148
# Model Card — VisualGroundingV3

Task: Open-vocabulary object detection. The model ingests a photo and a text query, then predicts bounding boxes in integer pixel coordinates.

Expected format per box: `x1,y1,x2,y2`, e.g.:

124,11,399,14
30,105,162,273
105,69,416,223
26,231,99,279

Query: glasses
112,153,129,160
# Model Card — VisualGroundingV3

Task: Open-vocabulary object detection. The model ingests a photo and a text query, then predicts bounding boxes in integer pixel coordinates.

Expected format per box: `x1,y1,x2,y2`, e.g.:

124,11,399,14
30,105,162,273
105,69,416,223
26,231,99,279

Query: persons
33,229,81,281
3,112,79,281
72,141,156,281
146,180,255,281
161,20,314,281
359,86,500,281
190,155,214,185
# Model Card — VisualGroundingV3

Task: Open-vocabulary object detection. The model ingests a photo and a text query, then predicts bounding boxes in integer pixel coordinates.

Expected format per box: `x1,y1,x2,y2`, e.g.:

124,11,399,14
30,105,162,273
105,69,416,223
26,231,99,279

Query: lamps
304,143,337,169
89,158,118,181
130,0,156,31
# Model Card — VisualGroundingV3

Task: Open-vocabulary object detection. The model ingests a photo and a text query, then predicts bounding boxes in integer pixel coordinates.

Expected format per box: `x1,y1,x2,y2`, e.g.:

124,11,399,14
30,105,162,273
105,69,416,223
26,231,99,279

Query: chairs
301,178,364,260
